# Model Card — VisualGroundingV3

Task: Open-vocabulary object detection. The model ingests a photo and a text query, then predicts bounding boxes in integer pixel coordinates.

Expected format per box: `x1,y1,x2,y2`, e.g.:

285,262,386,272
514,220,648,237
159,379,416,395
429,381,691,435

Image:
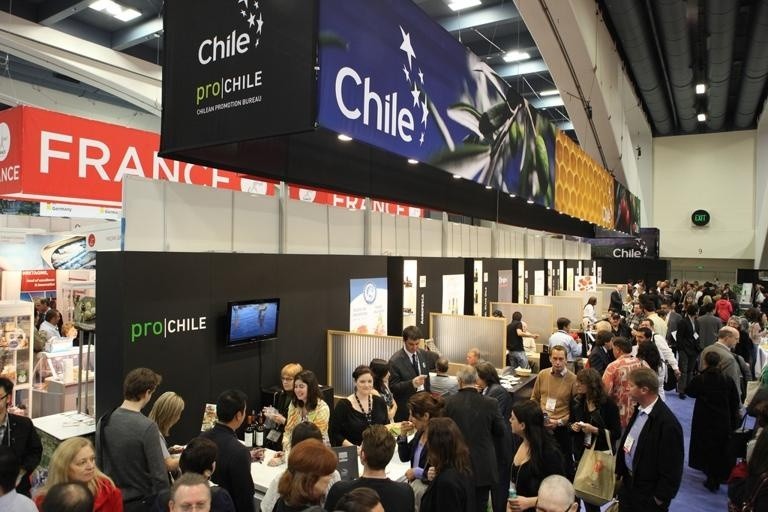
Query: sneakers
703,481,720,493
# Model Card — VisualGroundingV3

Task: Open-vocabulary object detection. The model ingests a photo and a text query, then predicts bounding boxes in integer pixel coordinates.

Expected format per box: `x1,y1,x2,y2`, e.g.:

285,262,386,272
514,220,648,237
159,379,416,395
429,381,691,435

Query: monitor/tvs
224,298,280,348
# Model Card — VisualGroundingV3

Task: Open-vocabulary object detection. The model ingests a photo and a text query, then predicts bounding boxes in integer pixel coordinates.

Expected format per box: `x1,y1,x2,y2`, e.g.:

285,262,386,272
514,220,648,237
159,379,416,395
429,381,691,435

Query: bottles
509,487,516,498
244,410,266,449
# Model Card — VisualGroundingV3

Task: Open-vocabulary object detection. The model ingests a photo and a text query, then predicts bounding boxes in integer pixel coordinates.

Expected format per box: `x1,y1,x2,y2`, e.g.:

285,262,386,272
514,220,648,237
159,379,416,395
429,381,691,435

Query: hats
610,312,621,319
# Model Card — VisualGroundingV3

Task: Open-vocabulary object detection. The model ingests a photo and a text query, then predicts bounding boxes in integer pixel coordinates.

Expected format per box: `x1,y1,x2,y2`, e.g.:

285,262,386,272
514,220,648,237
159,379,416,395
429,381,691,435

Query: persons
0,275,768,512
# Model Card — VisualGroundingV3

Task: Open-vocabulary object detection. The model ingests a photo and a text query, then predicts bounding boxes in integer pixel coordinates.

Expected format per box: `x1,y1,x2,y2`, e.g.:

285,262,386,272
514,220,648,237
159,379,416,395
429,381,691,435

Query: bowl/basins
514,368,532,376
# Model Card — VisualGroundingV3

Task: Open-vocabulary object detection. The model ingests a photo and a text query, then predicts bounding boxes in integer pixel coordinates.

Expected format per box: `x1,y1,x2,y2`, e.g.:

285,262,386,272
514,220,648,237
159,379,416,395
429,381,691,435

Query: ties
412,353,420,377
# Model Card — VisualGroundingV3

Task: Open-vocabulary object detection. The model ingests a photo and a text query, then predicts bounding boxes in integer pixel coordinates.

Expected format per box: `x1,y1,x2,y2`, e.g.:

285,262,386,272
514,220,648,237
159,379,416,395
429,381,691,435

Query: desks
753,334,768,377
167,324,612,512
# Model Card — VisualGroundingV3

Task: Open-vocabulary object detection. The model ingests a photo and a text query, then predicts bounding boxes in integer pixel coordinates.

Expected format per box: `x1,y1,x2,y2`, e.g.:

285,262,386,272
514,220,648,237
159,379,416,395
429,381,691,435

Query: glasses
533,497,573,512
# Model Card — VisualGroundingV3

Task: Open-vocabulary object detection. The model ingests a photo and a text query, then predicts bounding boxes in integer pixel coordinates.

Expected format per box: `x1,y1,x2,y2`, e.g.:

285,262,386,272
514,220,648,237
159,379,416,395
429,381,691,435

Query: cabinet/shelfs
0,300,34,419
28,343,95,418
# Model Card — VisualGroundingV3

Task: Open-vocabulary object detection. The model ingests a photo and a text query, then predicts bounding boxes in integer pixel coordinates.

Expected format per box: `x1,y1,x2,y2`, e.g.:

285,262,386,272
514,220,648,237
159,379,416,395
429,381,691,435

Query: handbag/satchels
743,367,767,409
727,472,768,512
571,428,617,508
733,411,755,459
